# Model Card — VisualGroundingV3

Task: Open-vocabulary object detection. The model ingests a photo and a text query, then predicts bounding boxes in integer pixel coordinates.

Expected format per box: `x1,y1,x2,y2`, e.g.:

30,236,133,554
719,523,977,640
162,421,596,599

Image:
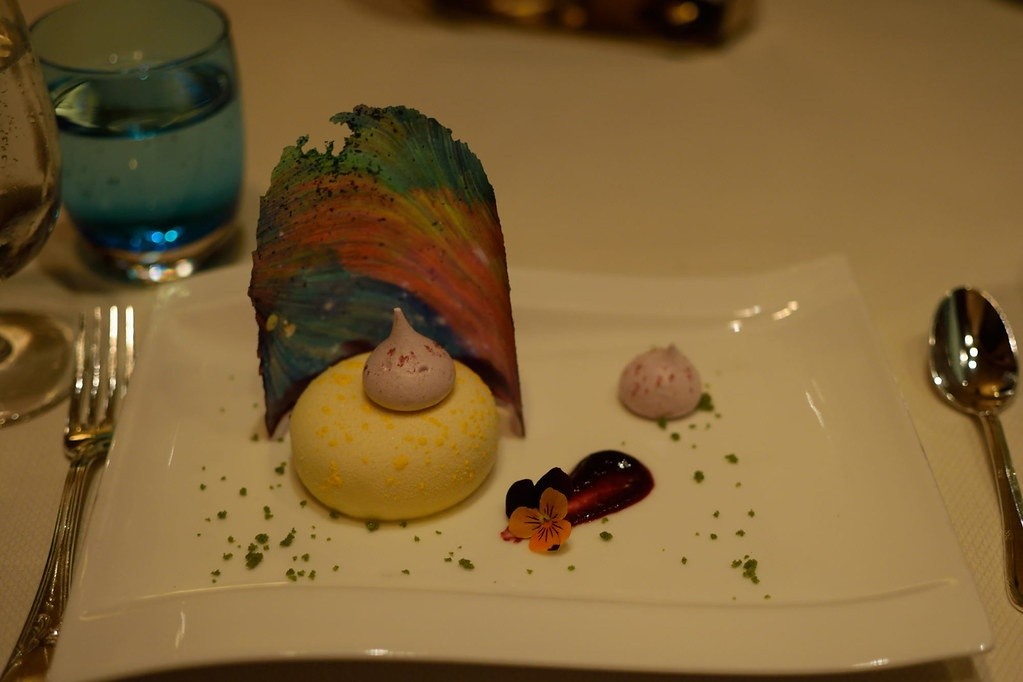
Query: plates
44,269,994,682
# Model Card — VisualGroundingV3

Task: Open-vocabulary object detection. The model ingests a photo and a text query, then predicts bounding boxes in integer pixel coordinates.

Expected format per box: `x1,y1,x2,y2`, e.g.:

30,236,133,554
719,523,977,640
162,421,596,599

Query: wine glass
0,0,78,424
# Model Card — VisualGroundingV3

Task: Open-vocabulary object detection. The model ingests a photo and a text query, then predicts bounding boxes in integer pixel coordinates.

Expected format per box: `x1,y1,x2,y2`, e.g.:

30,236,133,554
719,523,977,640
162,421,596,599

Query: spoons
927,283,1023,614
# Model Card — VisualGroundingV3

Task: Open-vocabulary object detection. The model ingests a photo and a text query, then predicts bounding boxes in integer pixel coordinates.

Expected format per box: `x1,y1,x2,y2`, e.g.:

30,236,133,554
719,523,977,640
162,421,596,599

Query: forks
0,303,135,681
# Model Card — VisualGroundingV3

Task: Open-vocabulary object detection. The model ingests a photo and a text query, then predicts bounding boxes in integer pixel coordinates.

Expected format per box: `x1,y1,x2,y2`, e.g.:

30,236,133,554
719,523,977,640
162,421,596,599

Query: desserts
245,98,528,521
619,346,703,424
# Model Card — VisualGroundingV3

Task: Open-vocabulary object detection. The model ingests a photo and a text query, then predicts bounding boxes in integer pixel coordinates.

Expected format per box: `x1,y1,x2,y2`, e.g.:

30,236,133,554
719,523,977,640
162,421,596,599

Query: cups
28,0,245,270
